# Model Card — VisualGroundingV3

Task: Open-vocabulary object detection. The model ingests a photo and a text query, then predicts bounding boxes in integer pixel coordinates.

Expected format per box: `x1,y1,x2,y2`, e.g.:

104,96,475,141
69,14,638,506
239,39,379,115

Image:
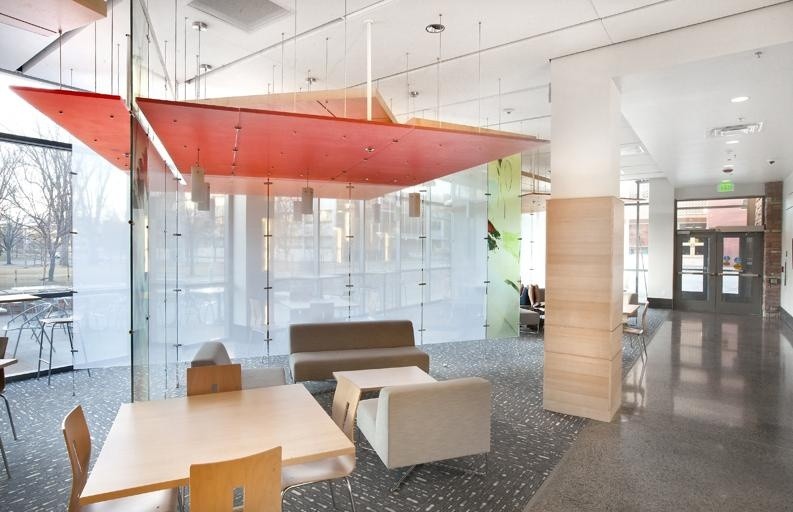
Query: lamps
190,145,206,207
293,188,305,223
409,179,422,219
303,160,315,218
197,180,211,216
372,194,382,225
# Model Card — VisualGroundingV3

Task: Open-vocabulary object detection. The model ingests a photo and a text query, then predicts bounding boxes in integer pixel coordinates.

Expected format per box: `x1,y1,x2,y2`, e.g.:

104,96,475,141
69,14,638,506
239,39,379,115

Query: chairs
517,280,652,356
